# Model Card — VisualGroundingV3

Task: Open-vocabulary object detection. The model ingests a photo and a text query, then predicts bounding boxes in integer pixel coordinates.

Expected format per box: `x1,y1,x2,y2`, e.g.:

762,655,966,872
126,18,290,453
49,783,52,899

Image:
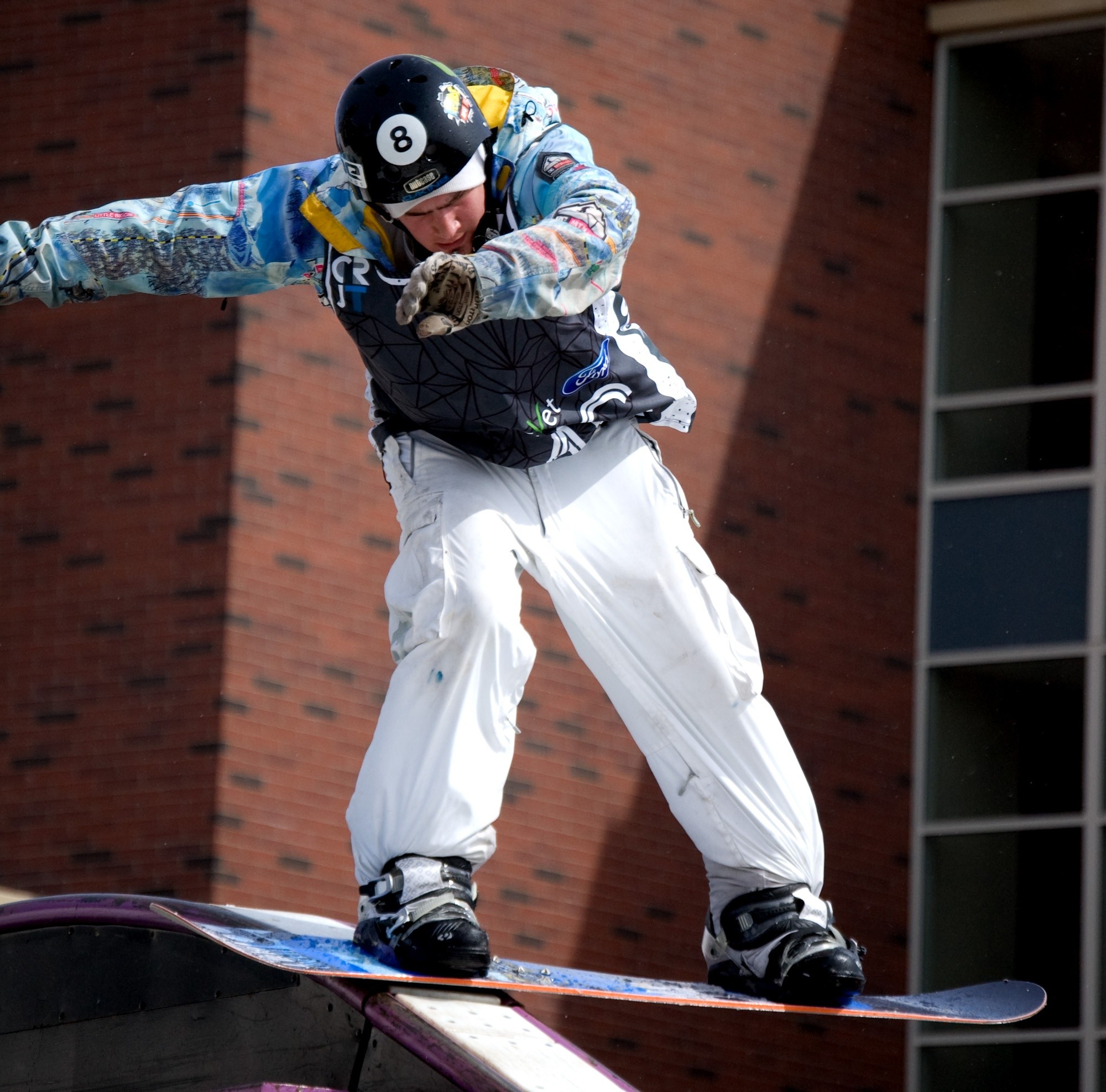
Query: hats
383,140,486,219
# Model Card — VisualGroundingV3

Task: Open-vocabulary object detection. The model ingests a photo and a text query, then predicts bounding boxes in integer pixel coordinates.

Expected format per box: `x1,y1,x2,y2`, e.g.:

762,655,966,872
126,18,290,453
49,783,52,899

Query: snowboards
151,900,1047,1027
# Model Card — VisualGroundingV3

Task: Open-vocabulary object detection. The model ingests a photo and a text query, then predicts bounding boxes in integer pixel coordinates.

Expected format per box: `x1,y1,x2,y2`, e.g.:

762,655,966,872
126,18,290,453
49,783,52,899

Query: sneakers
351,850,492,978
700,877,867,1003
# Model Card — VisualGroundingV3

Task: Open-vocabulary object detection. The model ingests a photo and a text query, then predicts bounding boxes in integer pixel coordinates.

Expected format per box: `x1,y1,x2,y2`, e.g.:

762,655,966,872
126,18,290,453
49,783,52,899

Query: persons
0,54,866,1010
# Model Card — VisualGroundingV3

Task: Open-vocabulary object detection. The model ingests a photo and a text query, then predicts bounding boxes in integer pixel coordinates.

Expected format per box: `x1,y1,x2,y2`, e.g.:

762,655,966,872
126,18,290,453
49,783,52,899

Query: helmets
333,55,494,206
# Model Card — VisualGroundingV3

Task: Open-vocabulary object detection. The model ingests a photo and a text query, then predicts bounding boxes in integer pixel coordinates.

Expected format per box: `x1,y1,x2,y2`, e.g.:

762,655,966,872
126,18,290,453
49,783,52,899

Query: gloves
394,251,482,340
